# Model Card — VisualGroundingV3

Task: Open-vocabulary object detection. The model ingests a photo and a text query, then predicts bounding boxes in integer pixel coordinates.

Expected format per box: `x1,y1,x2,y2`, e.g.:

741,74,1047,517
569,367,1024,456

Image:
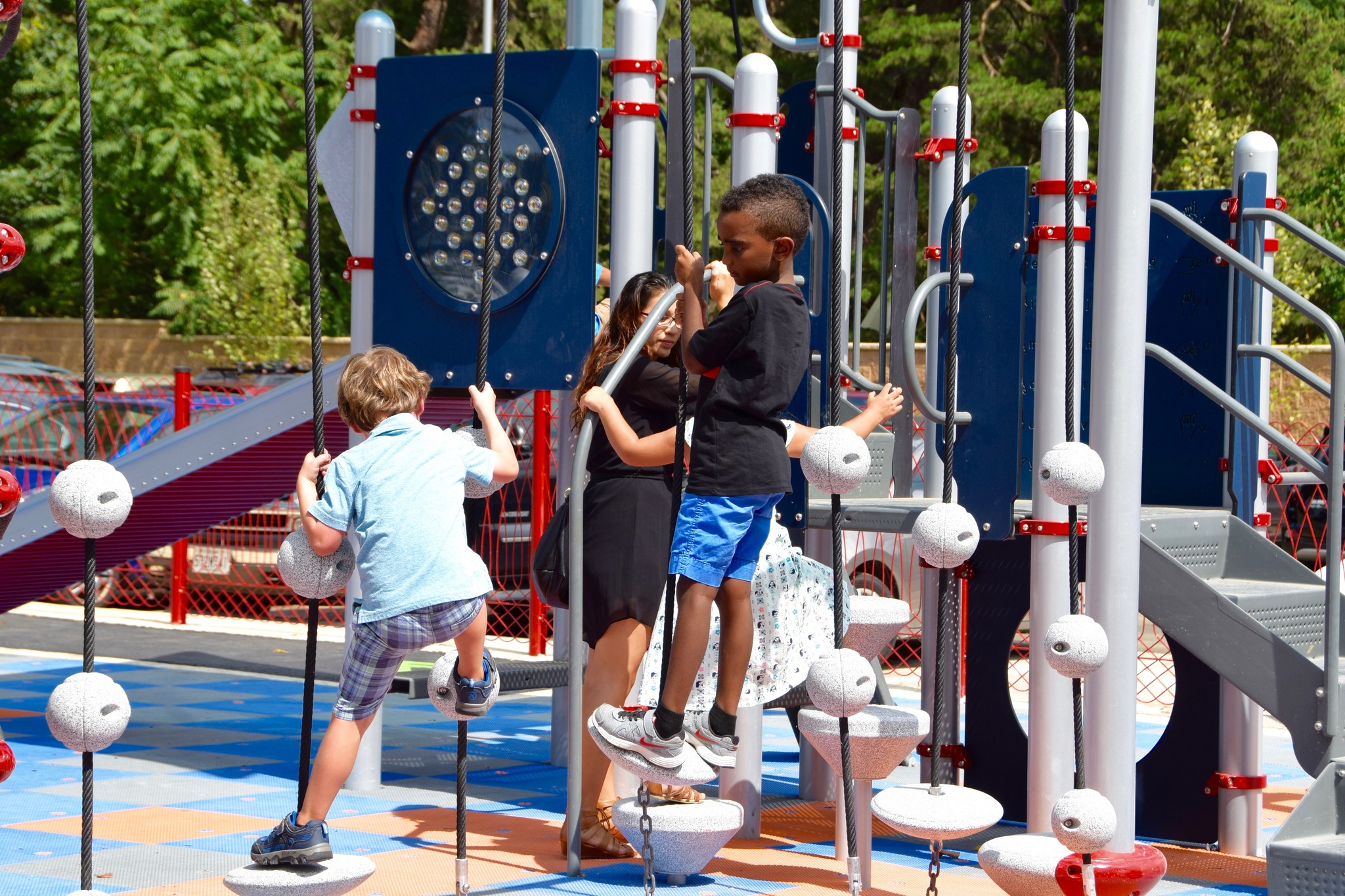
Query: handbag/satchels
532,492,571,609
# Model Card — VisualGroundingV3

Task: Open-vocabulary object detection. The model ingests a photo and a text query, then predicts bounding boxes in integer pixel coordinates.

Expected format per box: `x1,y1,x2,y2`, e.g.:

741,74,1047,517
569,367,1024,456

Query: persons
249,345,521,874
565,172,904,866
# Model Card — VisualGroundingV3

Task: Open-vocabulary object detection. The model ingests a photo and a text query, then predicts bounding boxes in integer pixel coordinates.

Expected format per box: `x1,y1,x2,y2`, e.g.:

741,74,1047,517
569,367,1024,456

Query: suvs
0,353,90,473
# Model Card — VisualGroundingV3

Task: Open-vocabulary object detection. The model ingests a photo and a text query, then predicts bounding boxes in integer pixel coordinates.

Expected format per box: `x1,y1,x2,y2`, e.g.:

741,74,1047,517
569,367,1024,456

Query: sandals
643,779,706,804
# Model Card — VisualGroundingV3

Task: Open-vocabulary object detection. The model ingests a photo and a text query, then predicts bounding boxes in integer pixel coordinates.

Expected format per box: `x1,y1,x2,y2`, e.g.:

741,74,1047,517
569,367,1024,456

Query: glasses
641,311,684,329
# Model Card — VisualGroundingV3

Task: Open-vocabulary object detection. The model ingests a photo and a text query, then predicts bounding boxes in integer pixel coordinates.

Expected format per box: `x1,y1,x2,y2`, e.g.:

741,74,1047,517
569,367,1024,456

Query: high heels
559,796,637,859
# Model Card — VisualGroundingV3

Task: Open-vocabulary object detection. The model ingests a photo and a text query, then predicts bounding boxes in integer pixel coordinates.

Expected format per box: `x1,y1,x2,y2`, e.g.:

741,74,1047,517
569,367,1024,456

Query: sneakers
681,710,739,768
451,643,496,717
592,703,687,768
250,810,333,865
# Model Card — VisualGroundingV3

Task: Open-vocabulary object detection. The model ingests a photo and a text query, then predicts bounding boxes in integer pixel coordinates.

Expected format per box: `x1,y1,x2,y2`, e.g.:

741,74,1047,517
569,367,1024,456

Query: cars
1267,427,1345,565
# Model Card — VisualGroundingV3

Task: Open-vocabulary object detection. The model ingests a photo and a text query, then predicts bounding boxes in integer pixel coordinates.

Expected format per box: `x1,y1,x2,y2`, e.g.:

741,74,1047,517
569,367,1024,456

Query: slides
1,354,351,616
